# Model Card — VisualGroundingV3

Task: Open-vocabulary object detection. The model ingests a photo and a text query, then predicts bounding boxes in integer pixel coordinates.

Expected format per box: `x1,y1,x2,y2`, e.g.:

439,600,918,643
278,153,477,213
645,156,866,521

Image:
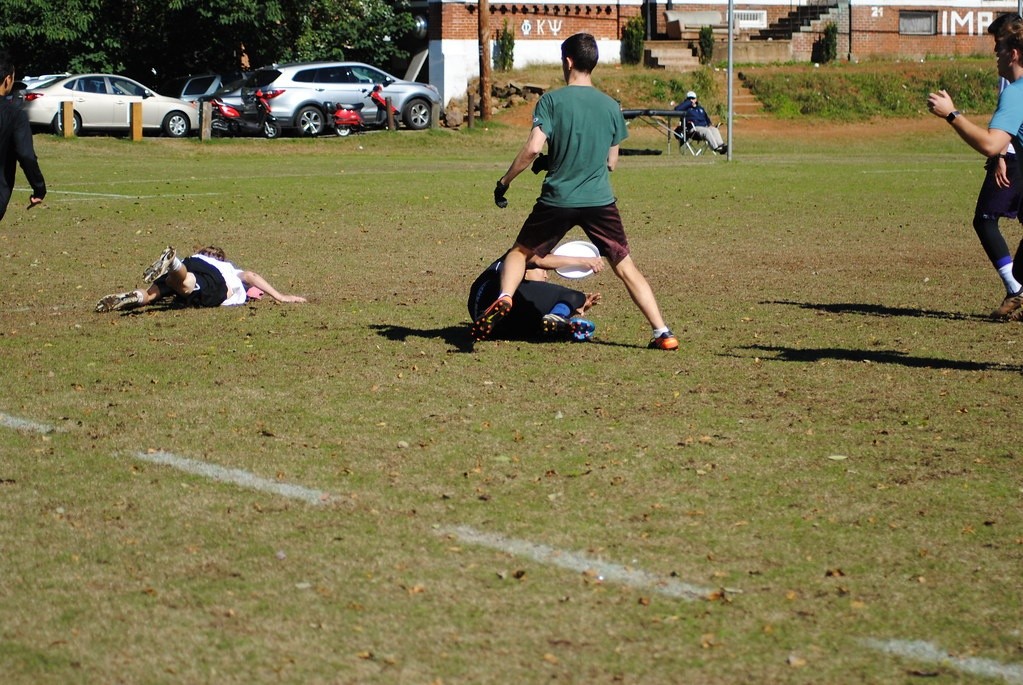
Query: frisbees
551,240,601,280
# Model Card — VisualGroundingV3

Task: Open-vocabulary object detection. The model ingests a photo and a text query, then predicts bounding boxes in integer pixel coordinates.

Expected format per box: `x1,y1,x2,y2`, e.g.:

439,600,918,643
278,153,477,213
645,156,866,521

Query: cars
5,72,200,137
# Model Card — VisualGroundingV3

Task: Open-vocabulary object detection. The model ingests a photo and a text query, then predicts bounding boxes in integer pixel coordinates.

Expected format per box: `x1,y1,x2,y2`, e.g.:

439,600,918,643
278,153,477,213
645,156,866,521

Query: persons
675,90,728,158
925,14,1023,322
0,58,47,223
468,34,682,351
95,245,306,313
467,247,605,342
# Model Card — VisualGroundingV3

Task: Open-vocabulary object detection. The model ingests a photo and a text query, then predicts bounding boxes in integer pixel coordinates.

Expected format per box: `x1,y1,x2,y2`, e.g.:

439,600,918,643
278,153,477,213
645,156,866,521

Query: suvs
179,61,443,138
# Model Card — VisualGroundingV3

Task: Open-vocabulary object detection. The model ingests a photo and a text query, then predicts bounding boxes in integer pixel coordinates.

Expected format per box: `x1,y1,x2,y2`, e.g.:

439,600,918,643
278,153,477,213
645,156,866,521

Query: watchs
997,153,1006,158
946,111,960,124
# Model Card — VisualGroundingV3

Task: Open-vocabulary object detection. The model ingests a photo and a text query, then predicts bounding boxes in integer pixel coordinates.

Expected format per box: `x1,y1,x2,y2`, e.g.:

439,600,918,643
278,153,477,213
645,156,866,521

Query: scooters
323,78,401,138
199,77,282,139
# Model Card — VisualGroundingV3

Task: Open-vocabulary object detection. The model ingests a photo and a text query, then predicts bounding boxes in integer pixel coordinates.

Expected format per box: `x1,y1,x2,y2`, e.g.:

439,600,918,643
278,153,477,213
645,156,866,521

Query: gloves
532,152,551,175
494,178,511,207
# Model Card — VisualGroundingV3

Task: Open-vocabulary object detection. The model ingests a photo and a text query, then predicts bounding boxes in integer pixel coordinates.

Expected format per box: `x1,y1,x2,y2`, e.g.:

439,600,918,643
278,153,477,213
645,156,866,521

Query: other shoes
140,245,176,284
714,143,728,155
542,314,595,337
92,291,141,313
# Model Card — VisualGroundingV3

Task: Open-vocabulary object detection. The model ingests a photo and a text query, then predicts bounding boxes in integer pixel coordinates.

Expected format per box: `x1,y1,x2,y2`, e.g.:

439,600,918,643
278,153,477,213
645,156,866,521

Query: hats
686,91,697,99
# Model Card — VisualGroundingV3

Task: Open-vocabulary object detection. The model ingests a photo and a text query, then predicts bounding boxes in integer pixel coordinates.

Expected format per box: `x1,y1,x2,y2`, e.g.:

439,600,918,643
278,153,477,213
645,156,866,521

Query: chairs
678,121,723,156
84,80,118,94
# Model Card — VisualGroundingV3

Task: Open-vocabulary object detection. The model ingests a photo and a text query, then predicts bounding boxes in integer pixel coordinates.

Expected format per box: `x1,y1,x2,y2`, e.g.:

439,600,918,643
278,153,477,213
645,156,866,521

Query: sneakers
989,285,1023,321
469,295,513,343
647,331,679,350
